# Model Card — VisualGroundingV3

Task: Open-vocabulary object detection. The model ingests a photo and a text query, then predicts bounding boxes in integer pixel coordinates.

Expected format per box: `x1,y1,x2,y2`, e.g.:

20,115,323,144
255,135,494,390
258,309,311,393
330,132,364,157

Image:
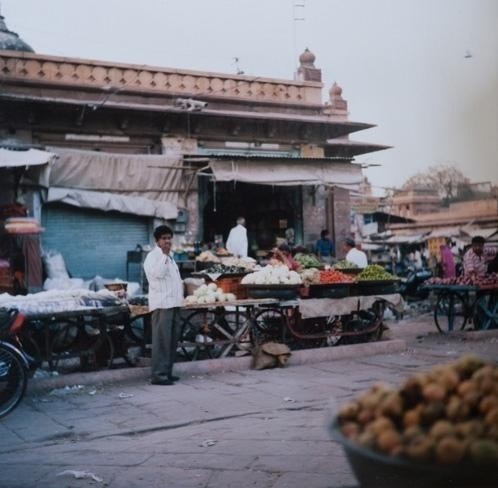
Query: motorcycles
388,255,432,301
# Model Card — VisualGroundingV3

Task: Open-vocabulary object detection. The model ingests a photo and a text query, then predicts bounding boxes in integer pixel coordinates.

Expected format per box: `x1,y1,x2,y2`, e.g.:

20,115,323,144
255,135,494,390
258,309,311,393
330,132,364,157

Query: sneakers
151,375,180,385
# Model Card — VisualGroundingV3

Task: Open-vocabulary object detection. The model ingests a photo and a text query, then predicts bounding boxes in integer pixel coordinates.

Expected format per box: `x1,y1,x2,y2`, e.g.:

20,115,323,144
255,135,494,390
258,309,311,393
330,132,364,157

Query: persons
344,237,369,270
144,224,185,386
422,248,432,267
464,235,498,283
226,217,253,261
439,243,456,279
316,230,338,265
413,247,423,269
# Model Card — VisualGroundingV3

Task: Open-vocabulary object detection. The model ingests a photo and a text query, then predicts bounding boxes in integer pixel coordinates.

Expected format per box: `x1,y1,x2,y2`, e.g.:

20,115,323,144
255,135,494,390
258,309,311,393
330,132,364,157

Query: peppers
356,264,394,281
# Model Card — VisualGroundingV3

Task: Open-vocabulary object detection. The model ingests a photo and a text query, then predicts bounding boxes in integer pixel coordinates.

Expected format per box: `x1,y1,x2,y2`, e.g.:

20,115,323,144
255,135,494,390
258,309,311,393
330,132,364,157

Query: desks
0,251,498,375
